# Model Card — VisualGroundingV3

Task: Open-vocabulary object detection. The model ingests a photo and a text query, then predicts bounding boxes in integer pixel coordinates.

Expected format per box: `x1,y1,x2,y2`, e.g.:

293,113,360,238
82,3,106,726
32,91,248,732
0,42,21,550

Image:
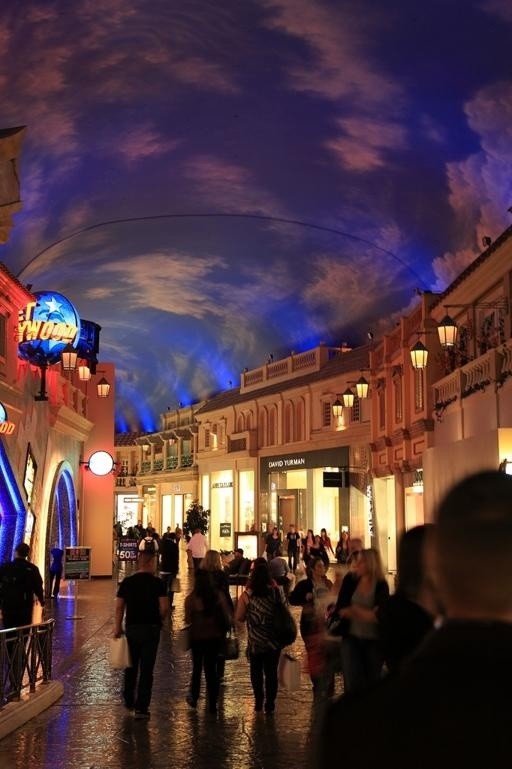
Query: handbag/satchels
271,602,297,648
278,653,299,693
178,627,189,649
108,635,128,666
227,635,237,662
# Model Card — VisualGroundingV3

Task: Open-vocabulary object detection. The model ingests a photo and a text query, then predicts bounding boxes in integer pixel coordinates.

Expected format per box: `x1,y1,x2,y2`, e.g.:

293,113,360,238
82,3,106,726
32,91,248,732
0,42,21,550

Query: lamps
356,364,401,401
168,435,176,447
63,342,111,399
410,326,466,369
332,394,343,419
437,297,508,346
342,381,379,408
142,439,149,452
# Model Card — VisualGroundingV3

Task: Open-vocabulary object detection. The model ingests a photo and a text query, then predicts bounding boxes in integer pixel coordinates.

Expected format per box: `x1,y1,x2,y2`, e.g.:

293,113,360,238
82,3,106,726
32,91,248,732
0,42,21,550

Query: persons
306,468,511,767
46,540,65,599
336,547,389,698
234,556,285,714
1,543,45,687
186,568,234,714
112,548,171,718
347,521,449,698
200,550,233,685
113,523,364,611
287,554,339,704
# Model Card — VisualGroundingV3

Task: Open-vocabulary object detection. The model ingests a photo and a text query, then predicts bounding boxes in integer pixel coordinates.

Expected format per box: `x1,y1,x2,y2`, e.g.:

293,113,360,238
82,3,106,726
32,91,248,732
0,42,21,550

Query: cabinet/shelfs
63,546,92,582
